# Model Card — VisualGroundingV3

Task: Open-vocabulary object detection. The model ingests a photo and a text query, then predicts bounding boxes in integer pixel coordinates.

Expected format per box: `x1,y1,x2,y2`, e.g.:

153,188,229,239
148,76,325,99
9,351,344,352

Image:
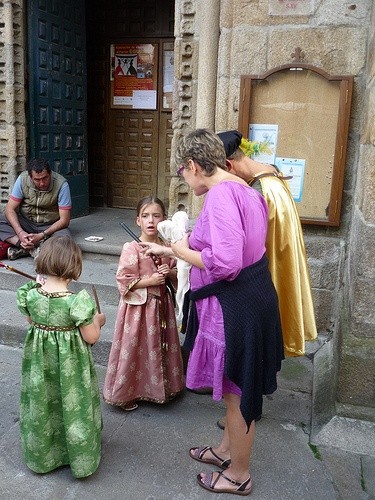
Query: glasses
175,158,191,176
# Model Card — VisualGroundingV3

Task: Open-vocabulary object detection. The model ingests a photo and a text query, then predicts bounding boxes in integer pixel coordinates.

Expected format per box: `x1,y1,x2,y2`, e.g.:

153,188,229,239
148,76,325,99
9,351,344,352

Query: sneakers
7,246,41,263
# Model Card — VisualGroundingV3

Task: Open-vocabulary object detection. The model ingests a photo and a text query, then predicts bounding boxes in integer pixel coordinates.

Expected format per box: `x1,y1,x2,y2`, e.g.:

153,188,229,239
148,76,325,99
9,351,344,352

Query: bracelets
41,230,48,240
17,228,25,236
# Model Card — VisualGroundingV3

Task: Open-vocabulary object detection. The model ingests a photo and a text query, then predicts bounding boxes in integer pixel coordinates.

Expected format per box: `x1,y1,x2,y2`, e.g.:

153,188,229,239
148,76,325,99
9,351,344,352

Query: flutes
120,221,176,308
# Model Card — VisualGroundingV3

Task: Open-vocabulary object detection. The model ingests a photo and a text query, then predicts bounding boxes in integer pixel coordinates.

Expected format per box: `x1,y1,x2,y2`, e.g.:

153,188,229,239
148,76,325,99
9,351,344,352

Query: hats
216,129,243,159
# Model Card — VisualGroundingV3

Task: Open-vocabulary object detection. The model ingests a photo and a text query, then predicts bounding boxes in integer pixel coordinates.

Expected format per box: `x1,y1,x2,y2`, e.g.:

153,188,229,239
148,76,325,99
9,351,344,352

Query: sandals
190,444,252,496
117,401,139,411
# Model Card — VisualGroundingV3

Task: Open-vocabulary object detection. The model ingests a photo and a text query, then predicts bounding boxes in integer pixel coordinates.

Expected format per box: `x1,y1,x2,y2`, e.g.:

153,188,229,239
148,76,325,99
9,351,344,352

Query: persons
137,127,286,497
184,130,318,430
15,235,106,479
102,195,186,411
0,157,74,262
